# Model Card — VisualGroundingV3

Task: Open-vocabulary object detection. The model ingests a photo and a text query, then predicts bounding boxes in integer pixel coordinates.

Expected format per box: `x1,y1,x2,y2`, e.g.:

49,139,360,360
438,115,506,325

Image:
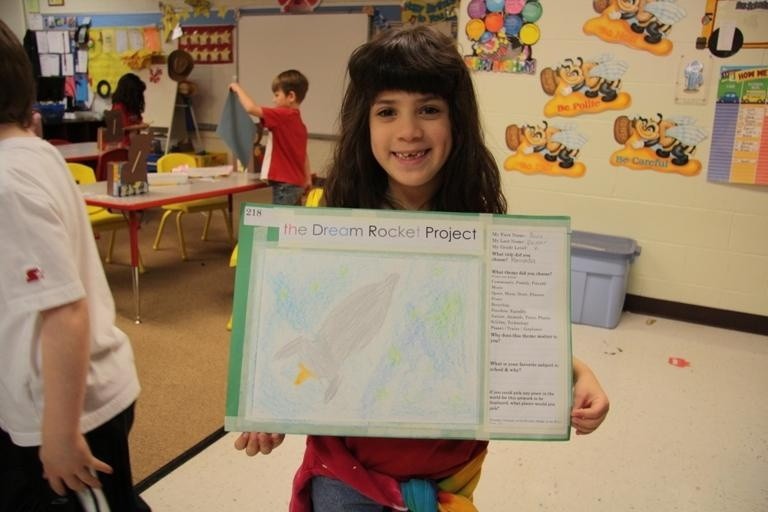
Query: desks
55,141,115,162
79,171,273,324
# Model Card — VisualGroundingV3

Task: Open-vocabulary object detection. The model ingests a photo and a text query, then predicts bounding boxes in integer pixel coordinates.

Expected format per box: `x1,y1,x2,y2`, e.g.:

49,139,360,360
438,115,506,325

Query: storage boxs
570,232,641,329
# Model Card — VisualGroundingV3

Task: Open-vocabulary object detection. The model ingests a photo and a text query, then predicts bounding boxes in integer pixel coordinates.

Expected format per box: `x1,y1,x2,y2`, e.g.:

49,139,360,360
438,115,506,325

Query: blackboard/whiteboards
235,11,371,142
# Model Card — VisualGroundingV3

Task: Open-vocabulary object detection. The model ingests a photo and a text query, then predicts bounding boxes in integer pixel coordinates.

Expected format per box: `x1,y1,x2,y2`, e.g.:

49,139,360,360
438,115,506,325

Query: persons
112,73,149,151
229,70,312,205
235,24,608,512
0,19,153,512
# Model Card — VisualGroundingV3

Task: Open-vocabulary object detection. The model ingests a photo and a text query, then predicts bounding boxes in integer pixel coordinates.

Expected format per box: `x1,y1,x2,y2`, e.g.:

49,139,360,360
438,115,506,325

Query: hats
168,50,193,82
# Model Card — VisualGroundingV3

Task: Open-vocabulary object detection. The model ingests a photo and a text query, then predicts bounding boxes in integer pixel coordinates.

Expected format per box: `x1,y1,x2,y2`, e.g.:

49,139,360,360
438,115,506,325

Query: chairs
153,151,233,260
66,162,145,275
